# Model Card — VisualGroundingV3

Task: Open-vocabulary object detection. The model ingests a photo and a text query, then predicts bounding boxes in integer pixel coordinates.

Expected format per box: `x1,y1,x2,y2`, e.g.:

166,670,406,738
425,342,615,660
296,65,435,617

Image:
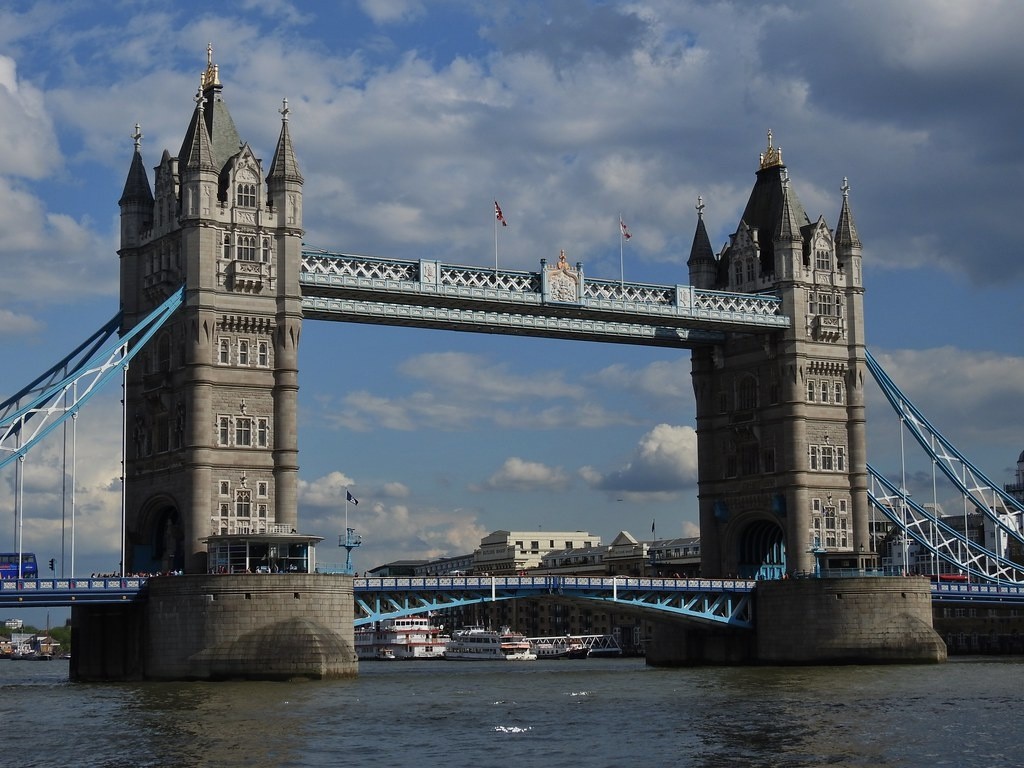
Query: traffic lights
49,559,54,570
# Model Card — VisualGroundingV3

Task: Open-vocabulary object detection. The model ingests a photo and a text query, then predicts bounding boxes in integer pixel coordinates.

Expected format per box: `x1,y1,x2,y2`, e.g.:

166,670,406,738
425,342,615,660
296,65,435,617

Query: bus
449,570,466,576
924,574,977,584
0,552,38,578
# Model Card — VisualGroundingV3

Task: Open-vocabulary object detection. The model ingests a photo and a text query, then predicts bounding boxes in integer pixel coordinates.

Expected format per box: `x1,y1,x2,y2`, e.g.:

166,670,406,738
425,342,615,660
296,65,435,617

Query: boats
499,625,538,661
444,616,506,661
537,640,593,660
10,641,38,661
31,634,61,657
59,653,72,659
355,616,456,660
0,641,17,658
376,648,396,660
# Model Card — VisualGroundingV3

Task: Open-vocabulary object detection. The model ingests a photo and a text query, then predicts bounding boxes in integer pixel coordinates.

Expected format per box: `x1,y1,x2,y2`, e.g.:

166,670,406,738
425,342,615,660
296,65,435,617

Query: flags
495,202,507,226
652,522,655,532
347,491,358,506
621,221,630,240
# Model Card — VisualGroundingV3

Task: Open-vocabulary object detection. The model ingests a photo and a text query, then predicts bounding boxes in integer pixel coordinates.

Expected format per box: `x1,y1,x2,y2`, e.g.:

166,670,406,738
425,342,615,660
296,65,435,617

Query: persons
364,572,366,577
208,565,233,574
91,568,182,578
355,573,358,577
246,564,278,573
672,573,680,578
520,570,528,574
